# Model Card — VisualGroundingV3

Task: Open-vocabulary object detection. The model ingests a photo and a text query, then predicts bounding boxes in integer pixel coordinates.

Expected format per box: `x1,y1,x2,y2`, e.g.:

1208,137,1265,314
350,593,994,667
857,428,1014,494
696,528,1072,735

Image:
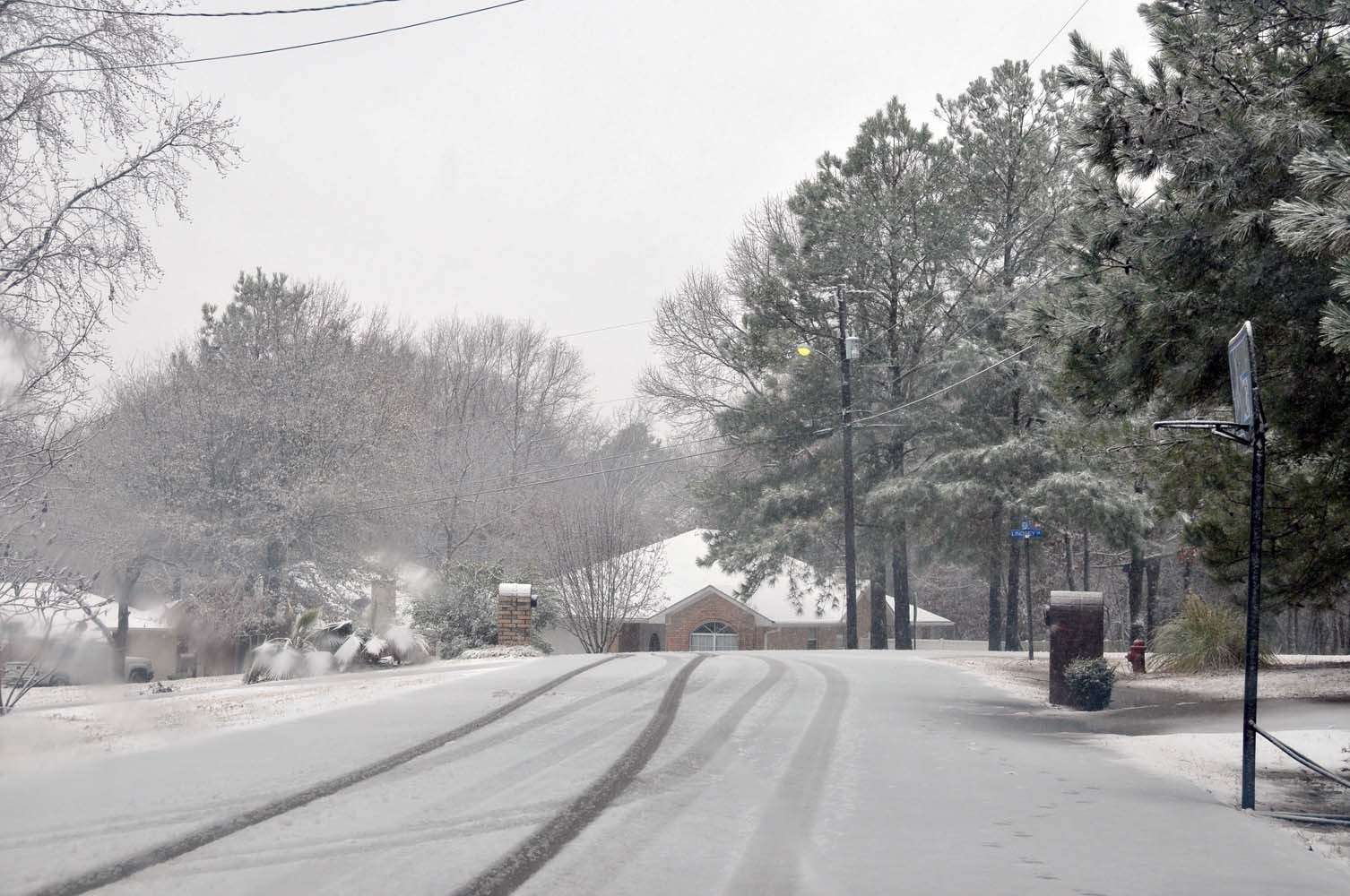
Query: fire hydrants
1125,638,1147,673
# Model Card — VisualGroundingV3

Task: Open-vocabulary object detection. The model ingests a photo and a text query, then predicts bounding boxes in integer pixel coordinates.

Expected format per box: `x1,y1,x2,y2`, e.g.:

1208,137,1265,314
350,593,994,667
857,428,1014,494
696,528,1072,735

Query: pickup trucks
3,651,154,685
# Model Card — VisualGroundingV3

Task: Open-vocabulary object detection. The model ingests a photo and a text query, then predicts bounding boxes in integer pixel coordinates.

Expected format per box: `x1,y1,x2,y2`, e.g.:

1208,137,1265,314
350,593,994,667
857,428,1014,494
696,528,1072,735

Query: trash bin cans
1044,591,1104,706
